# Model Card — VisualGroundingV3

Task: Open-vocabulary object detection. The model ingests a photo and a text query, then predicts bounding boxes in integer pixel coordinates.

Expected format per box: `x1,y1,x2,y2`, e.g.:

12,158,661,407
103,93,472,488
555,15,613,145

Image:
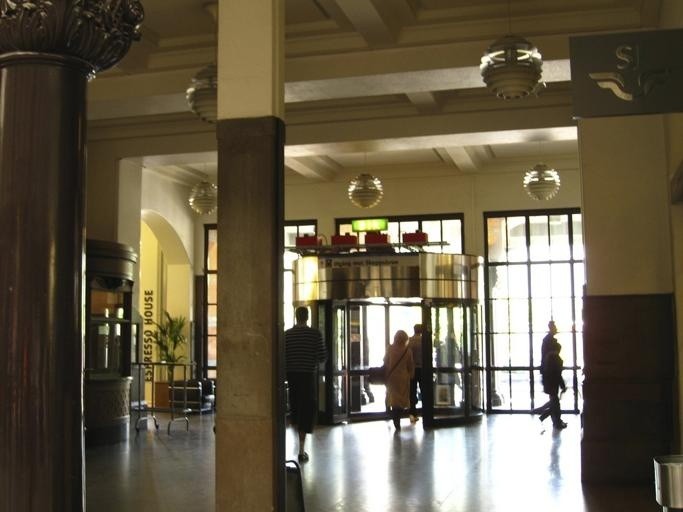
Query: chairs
298,452,309,464
554,420,568,429
409,413,420,424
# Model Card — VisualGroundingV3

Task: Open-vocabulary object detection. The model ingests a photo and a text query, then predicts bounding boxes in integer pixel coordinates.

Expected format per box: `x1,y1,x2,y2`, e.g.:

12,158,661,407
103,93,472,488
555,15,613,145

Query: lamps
188,380,215,408
169,380,212,414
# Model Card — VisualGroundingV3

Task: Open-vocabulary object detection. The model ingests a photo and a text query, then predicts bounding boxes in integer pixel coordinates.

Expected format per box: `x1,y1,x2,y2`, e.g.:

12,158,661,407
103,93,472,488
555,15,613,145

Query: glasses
190,164,218,216
348,152,384,208
185,60,214,122
478,0,543,101
522,141,560,200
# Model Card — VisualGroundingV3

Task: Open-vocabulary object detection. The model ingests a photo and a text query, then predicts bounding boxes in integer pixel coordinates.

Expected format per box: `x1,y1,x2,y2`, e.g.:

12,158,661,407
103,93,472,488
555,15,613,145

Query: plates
131,362,197,435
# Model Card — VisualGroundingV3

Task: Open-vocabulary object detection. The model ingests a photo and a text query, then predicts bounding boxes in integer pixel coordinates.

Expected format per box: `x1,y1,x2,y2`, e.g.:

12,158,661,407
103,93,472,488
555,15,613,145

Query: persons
536,321,568,429
381,330,414,431
408,323,424,423
284,307,329,463
